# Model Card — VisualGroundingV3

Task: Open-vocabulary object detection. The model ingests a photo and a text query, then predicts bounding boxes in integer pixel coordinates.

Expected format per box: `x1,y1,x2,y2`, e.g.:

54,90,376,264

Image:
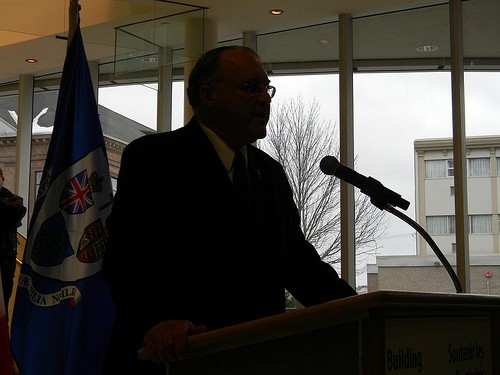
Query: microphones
320,156,410,211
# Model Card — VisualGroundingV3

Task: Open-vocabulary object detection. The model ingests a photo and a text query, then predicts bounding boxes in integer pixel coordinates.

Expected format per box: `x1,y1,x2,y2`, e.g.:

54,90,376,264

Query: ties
232,151,252,202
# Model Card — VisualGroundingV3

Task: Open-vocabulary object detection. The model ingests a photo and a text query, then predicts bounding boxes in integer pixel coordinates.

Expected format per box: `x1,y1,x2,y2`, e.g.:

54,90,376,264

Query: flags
0,0,115,375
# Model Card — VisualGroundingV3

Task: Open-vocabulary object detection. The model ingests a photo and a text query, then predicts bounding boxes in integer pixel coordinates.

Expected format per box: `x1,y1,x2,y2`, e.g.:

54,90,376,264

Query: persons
101,46,357,375
0,167,27,322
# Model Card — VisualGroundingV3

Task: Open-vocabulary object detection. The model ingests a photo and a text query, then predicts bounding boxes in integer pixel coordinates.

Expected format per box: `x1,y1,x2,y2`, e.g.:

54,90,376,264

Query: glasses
230,84,276,98
